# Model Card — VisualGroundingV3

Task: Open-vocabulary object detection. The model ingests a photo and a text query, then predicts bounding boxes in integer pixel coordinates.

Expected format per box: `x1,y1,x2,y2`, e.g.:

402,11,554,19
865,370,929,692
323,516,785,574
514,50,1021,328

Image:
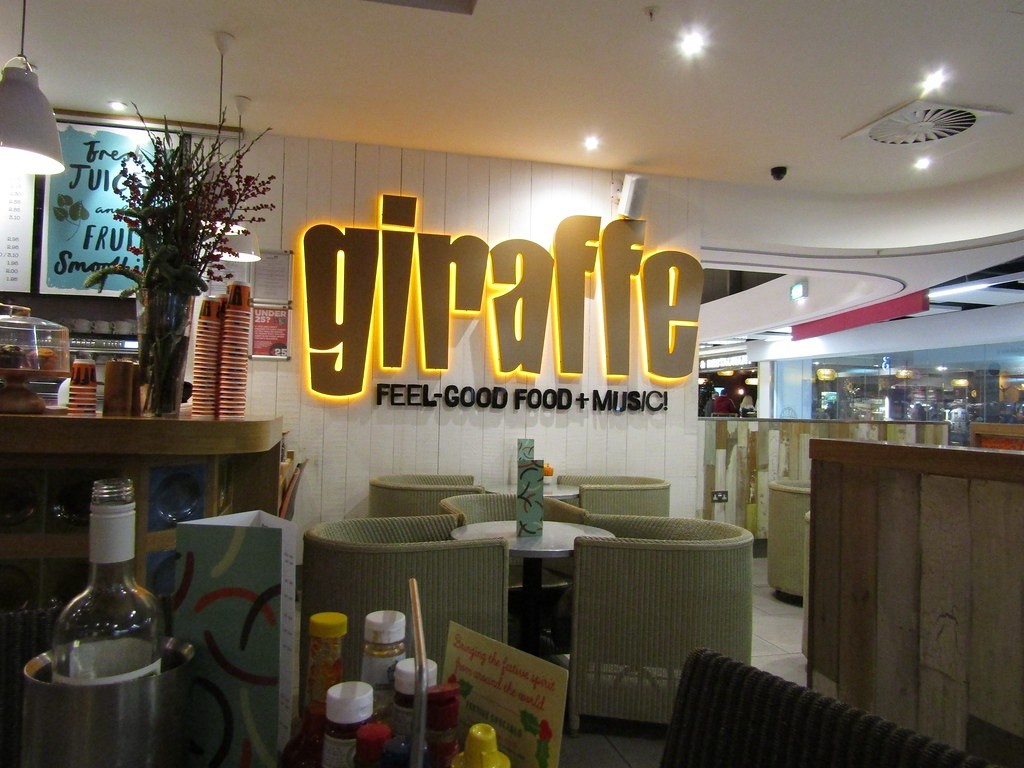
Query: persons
739,395,754,418
911,403,926,421
705,392,719,417
1009,407,1023,423
714,390,736,417
825,401,834,412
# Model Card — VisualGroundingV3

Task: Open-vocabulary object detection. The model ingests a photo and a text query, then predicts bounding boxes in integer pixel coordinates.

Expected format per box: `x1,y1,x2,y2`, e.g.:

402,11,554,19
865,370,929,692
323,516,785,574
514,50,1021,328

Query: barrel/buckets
20,638,198,767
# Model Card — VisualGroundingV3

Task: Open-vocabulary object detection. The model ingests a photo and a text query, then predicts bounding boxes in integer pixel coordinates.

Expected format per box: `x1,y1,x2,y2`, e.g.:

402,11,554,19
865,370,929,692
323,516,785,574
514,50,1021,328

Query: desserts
0,344,59,370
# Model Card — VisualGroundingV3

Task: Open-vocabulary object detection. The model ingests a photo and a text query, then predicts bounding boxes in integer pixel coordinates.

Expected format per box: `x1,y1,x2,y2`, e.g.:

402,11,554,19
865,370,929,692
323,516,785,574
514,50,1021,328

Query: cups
66,357,100,418
191,280,254,419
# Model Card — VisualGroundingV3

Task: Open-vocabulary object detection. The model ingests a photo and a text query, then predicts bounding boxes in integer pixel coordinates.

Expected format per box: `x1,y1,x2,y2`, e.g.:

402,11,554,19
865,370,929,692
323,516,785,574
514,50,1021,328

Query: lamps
198,30,248,236
0,0,66,176
213,96,261,262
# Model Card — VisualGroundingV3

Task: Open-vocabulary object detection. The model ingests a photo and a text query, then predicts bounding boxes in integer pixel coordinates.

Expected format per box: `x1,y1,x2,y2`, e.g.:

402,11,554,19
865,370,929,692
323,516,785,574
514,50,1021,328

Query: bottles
273,609,512,768
50,475,163,685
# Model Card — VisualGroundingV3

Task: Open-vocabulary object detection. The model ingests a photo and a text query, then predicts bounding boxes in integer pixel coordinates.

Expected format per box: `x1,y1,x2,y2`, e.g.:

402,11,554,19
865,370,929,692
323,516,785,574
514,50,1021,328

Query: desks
449,519,617,656
485,484,579,501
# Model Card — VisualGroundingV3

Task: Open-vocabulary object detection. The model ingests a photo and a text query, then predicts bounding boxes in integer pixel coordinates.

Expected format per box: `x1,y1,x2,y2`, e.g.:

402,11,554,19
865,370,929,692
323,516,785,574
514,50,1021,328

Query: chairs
367,475,486,518
570,511,755,740
556,474,671,517
660,645,991,768
766,479,811,605
436,494,589,650
299,513,511,721
